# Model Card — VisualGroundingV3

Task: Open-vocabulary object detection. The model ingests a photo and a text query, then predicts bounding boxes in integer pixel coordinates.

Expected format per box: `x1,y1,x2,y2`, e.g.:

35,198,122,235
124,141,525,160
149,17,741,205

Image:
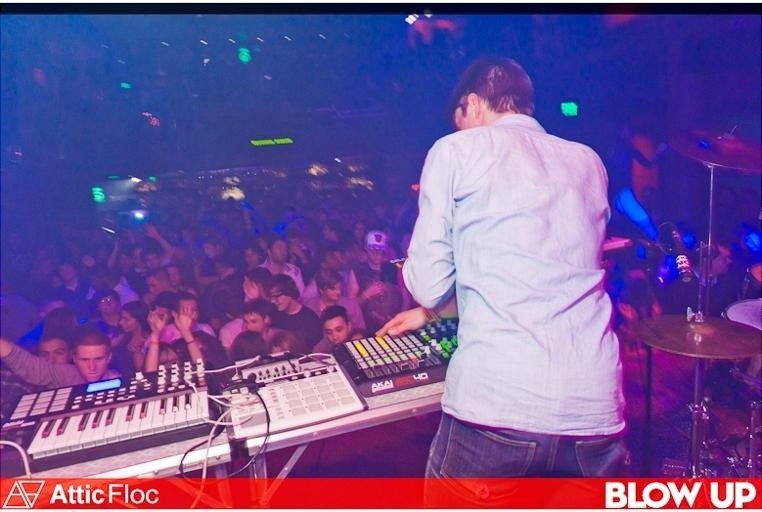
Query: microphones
657,221,693,283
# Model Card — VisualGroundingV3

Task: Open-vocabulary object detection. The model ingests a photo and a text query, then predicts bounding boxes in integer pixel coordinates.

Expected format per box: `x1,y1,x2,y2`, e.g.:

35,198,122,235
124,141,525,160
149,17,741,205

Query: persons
374,53,632,508
0,163,761,508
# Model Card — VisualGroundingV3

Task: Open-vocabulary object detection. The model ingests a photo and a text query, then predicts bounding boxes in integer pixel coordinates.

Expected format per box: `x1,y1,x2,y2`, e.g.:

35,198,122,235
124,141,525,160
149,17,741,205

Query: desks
3,428,240,509
236,390,445,509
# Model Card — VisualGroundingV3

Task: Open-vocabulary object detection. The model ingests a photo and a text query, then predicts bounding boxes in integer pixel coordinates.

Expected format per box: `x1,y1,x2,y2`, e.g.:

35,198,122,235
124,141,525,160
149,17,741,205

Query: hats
364,230,388,252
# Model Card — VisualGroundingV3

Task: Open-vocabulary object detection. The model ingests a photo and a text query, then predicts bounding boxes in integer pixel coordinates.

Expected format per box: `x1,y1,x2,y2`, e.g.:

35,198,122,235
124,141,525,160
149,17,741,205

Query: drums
737,262,762,302
724,298,762,388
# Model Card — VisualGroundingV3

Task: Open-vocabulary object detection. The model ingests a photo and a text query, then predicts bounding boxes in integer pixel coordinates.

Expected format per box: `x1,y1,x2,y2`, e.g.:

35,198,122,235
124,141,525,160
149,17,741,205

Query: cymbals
669,126,762,173
634,313,760,360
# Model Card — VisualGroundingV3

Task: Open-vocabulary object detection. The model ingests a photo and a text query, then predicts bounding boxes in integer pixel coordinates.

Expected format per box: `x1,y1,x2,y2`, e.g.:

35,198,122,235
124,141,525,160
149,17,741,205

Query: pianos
2,358,214,477
